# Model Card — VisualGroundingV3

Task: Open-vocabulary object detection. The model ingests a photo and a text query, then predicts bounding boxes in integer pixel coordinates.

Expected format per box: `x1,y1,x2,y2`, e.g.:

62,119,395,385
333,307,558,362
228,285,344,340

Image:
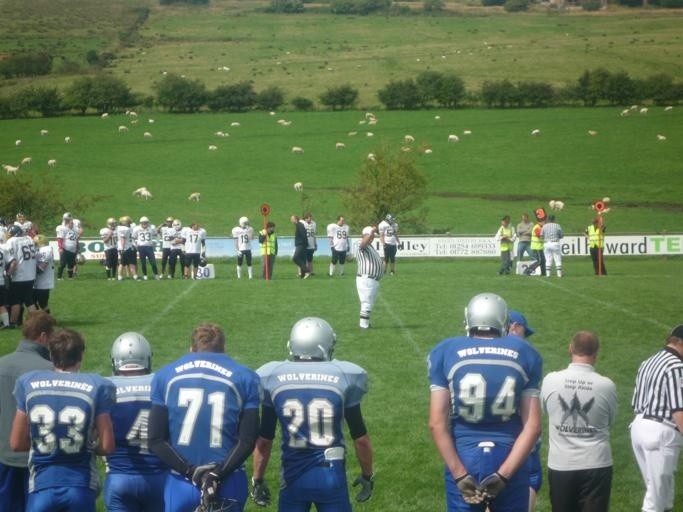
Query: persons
100,216,207,281
327,215,350,276
379,215,400,275
426,292,544,512
483,311,543,512
583,218,607,275
147,323,265,512
259,222,278,280
354,226,383,328
250,316,373,512
56,212,85,280
495,208,565,278
0,212,55,329
539,331,619,512
291,213,317,279
232,217,254,279
0,310,57,512
101,331,169,512
10,329,117,512
628,325,683,512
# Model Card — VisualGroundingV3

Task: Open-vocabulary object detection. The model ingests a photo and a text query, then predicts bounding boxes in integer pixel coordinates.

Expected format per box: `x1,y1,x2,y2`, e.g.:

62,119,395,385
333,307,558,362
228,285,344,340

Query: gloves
475,471,510,503
383,243,391,251
454,471,489,505
199,471,224,512
185,461,218,488
351,472,374,504
250,477,273,508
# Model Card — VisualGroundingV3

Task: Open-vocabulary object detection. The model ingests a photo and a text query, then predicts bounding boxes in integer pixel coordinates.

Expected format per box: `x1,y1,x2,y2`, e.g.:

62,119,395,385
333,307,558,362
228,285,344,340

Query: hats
671,324,683,342
548,215,555,220
301,211,311,219
505,310,536,337
502,215,510,221
362,226,378,236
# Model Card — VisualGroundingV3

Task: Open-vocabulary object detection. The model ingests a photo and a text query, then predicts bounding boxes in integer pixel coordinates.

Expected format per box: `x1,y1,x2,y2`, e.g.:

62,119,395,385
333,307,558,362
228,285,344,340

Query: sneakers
390,270,395,276
495,269,565,278
327,271,344,277
143,275,148,281
296,272,316,280
167,272,174,279
152,272,164,280
183,274,189,279
107,277,115,280
0,322,16,329
127,272,142,282
117,275,122,280
57,272,79,281
191,274,201,280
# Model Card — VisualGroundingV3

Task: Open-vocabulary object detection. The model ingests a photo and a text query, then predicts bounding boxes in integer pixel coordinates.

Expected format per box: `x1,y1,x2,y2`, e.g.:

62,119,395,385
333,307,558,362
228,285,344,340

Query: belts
643,414,680,433
316,460,345,467
356,273,378,281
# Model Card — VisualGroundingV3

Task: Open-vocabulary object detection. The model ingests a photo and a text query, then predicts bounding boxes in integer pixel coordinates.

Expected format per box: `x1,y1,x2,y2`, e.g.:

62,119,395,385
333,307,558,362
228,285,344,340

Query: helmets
239,216,249,226
63,212,73,220
109,331,152,377
385,213,396,221
166,216,173,222
75,253,86,266
106,217,117,228
118,216,133,226
172,219,182,231
139,216,149,223
6,225,23,237
33,234,49,247
286,316,337,362
463,292,509,337
199,257,207,268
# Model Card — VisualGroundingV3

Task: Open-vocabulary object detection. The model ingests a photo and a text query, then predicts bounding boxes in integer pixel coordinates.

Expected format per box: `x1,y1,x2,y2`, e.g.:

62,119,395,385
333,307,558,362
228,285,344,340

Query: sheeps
3,129,72,176
531,129,541,136
216,131,224,136
587,129,597,137
293,181,303,192
140,190,154,201
269,109,292,128
291,146,304,155
186,192,202,203
229,121,240,127
132,186,148,196
656,133,668,141
617,103,674,119
208,144,217,150
548,199,565,212
334,111,473,163
101,109,157,140
